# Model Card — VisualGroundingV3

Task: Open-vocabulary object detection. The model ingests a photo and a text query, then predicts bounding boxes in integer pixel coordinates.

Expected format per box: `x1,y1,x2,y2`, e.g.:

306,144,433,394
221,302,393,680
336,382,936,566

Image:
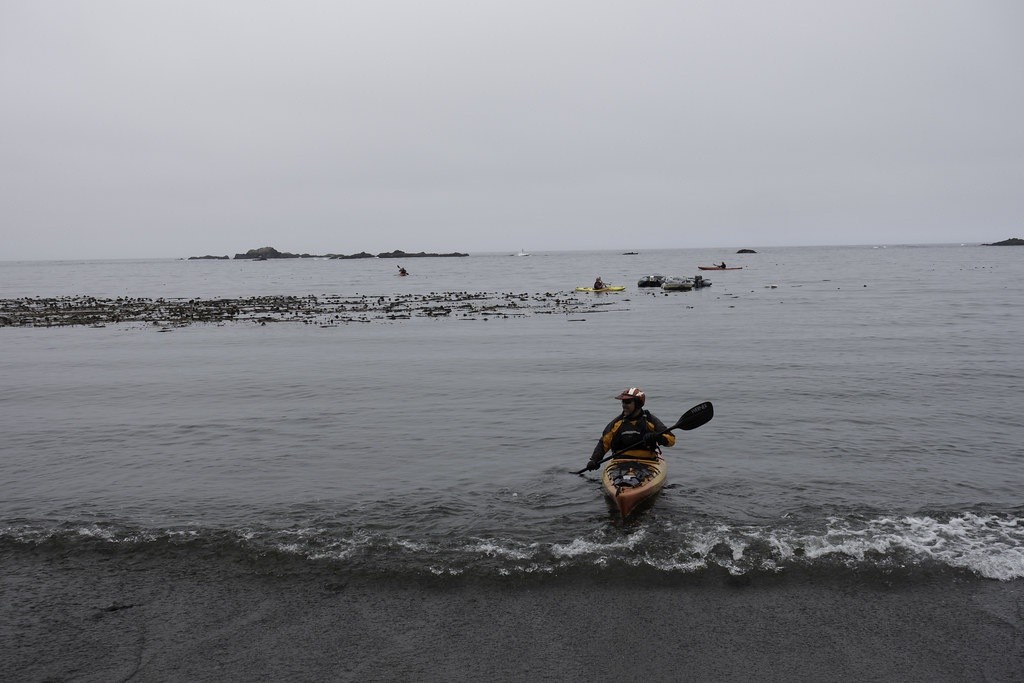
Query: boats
601,451,668,521
510,249,530,256
393,273,409,276
698,266,742,270
574,286,625,291
638,275,712,290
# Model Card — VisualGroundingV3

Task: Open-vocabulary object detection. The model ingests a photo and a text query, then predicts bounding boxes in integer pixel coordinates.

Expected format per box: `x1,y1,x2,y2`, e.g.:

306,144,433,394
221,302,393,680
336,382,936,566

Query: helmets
615,387,646,404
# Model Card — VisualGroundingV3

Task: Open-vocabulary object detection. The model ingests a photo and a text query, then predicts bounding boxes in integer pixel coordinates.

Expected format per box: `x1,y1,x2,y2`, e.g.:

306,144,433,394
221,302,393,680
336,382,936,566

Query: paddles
575,401,714,474
601,281,607,288
713,264,720,267
397,265,409,275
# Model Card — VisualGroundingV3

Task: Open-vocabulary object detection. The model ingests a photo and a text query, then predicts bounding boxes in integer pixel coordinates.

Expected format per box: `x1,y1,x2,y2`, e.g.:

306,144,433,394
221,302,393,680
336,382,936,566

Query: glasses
621,399,635,404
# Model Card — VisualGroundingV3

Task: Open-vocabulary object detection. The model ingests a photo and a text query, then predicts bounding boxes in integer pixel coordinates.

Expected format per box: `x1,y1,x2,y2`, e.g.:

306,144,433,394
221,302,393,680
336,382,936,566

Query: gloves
644,431,656,442
587,460,601,472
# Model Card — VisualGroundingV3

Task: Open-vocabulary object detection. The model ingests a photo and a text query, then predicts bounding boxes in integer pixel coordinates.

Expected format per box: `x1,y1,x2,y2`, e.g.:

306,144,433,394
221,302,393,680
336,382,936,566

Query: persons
594,277,603,290
587,388,676,472
717,261,726,268
399,267,406,272
